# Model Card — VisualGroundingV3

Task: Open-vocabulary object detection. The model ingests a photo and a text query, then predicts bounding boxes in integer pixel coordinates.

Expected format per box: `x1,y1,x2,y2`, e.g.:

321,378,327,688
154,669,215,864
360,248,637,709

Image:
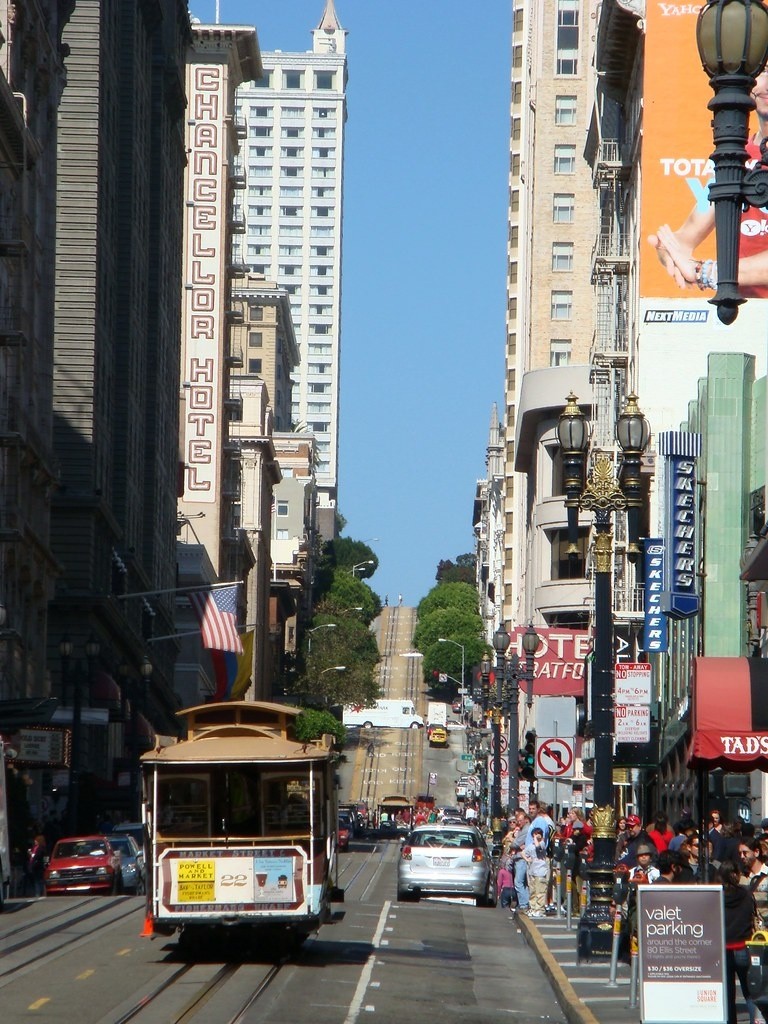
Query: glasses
509,821,516,824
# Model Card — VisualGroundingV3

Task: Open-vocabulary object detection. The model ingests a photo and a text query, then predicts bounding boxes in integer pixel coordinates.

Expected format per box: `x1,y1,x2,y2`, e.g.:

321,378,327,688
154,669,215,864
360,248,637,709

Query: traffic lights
525,731,535,766
518,749,534,780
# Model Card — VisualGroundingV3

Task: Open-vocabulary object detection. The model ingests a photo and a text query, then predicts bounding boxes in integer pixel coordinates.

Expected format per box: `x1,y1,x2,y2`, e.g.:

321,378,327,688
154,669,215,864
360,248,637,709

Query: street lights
321,666,346,703
345,607,363,612
438,638,464,715
364,538,379,542
347,567,365,574
58,632,101,834
494,621,539,817
353,560,374,577
308,624,336,653
695,0,768,326
481,651,508,853
118,655,153,824
555,390,652,966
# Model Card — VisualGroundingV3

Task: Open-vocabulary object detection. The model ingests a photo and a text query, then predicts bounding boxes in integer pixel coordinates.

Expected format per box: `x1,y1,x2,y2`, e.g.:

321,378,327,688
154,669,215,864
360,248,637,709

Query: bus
139,700,346,944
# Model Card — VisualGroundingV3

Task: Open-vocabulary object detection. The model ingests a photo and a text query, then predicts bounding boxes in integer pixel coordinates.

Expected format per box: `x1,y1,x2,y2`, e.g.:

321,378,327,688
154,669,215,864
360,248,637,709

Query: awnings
684,657,768,771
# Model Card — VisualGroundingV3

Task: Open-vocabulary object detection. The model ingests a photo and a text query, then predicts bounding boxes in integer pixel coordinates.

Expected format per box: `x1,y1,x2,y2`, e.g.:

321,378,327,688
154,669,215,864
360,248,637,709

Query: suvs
75,834,144,895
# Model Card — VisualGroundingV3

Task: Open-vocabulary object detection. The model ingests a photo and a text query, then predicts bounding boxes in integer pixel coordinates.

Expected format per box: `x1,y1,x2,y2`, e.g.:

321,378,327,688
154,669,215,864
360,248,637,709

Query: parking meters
553,833,564,920
626,872,649,1009
605,864,630,986
563,838,577,930
578,847,590,919
745,931,768,1024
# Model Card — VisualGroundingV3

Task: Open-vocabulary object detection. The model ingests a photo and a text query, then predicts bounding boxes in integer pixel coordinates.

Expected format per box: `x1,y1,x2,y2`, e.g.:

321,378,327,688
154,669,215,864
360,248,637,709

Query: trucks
376,796,414,832
342,700,424,730
424,702,449,729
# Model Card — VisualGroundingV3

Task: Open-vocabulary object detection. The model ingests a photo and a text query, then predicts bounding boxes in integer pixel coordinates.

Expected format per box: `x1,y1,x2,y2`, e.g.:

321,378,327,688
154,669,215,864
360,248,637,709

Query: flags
189,586,244,654
214,630,254,701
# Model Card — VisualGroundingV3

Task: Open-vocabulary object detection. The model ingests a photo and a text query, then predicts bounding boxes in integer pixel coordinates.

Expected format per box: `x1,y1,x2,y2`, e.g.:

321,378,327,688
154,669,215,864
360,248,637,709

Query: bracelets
696,259,715,291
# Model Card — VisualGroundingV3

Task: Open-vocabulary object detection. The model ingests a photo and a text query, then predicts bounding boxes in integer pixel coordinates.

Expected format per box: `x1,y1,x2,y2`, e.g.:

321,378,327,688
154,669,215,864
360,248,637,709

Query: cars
436,806,467,825
337,801,368,853
398,824,498,908
446,720,466,730
42,836,122,896
426,724,446,740
413,793,437,809
429,729,450,748
452,699,462,712
112,823,146,848
454,776,481,802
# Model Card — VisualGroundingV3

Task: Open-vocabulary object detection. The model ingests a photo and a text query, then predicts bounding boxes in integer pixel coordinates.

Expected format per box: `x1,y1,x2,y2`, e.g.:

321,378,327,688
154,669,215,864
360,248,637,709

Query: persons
399,593,402,606
647,62,768,292
384,594,389,606
628,845,660,882
496,800,768,1024
27,836,46,895
364,806,449,830
668,820,689,851
271,804,302,831
618,815,656,862
521,827,547,917
466,797,489,835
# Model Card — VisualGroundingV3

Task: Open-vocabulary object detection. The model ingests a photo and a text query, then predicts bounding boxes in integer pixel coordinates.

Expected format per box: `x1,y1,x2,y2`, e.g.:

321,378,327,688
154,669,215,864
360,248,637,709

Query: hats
634,846,654,858
507,816,516,821
623,815,641,825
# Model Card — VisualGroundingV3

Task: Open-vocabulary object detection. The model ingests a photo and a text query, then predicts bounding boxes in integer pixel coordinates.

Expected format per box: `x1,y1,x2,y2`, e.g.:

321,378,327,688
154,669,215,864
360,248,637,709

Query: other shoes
528,910,545,917
524,909,533,914
